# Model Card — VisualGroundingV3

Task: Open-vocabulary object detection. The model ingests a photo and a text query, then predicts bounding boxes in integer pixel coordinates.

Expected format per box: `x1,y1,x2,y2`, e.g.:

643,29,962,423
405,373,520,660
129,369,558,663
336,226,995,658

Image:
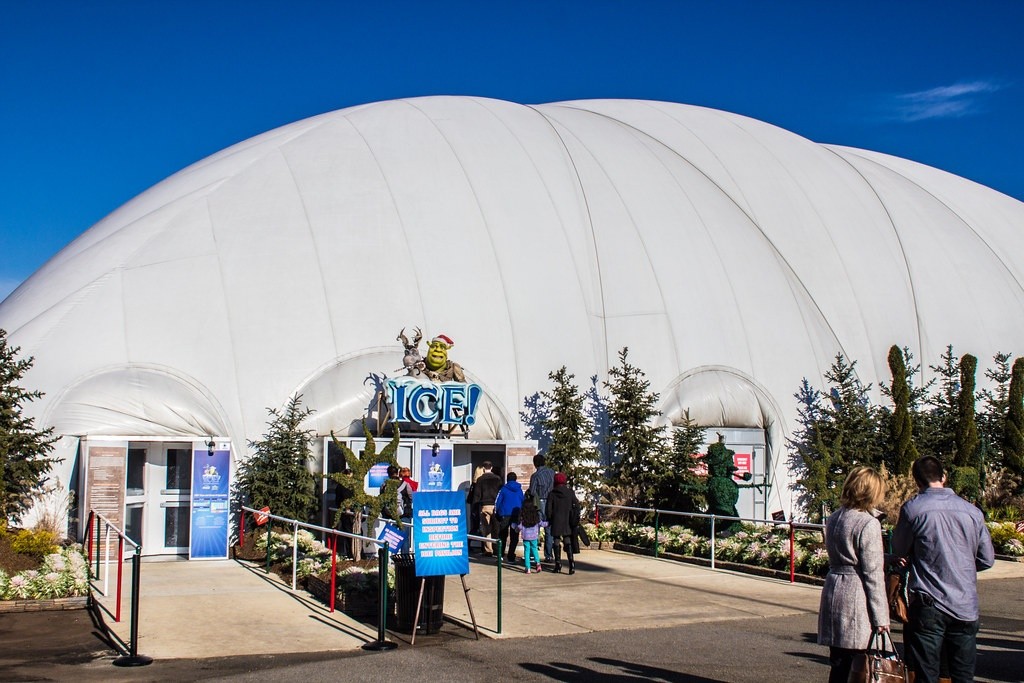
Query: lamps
208,435,215,456
434,436,440,456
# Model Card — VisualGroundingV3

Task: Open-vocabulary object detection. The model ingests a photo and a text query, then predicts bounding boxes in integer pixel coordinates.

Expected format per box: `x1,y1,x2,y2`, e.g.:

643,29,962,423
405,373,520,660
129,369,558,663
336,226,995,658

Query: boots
551,543,562,573
563,543,575,574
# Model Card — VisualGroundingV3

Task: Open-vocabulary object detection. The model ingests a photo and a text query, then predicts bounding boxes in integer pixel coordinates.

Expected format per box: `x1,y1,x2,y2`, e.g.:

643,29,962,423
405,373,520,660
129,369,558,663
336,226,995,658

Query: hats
553,473,566,484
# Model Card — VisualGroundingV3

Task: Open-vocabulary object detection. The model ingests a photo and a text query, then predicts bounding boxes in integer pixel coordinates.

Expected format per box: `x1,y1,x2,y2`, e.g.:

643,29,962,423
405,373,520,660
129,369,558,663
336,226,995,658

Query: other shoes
508,556,516,563
524,568,531,574
491,553,498,558
545,557,553,563
536,564,542,573
479,551,493,558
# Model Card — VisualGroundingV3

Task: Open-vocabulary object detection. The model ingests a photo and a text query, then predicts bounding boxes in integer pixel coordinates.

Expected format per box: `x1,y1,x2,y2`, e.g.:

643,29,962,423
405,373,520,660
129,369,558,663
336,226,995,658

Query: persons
511,504,549,576
492,468,524,564
380,466,418,565
525,453,556,562
466,460,504,559
817,465,916,683
546,468,580,576
891,454,995,683
336,469,367,559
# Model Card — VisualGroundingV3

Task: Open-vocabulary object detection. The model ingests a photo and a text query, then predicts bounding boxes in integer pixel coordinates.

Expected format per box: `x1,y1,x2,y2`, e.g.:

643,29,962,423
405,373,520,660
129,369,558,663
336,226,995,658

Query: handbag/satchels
884,570,909,624
861,627,908,683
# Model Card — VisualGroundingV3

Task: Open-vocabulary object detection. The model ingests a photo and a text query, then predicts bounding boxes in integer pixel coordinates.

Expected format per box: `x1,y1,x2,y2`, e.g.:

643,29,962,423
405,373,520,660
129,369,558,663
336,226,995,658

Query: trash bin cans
392,553,445,636
